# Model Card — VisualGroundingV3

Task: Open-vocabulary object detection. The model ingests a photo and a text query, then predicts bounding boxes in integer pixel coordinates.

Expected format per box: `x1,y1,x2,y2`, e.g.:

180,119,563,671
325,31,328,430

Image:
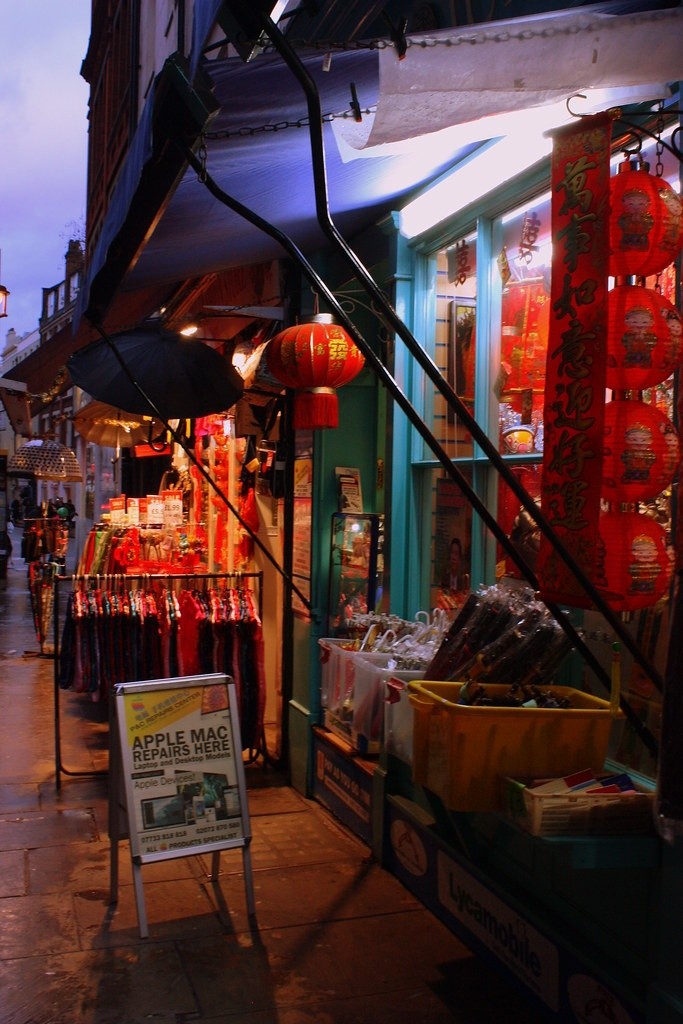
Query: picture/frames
447,299,477,425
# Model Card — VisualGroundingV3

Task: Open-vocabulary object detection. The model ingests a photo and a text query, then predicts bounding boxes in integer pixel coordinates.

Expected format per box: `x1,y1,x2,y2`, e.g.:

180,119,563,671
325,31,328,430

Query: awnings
0,0,683,440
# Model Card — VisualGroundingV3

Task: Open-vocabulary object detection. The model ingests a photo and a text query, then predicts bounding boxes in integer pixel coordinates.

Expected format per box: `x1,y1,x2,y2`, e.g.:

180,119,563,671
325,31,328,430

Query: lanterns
601,167,683,719
262,313,365,428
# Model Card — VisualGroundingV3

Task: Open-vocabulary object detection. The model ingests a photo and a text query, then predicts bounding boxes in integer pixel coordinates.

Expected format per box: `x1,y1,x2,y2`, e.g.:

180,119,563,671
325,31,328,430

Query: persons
337,522,370,627
48,497,76,529
441,538,466,592
11,485,45,580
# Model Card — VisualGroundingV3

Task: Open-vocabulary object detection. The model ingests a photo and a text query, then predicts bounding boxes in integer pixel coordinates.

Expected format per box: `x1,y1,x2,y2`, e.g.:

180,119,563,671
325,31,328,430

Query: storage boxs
311,637,683,914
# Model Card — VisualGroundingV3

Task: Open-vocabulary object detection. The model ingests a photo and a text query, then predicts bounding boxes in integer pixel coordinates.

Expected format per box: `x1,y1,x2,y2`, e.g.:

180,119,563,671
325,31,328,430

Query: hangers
71,571,244,592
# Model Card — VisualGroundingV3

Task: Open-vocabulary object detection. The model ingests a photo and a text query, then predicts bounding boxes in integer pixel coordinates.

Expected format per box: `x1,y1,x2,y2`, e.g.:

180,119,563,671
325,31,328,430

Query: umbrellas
28,561,59,653
64,325,244,451
330,607,447,713
73,404,167,483
